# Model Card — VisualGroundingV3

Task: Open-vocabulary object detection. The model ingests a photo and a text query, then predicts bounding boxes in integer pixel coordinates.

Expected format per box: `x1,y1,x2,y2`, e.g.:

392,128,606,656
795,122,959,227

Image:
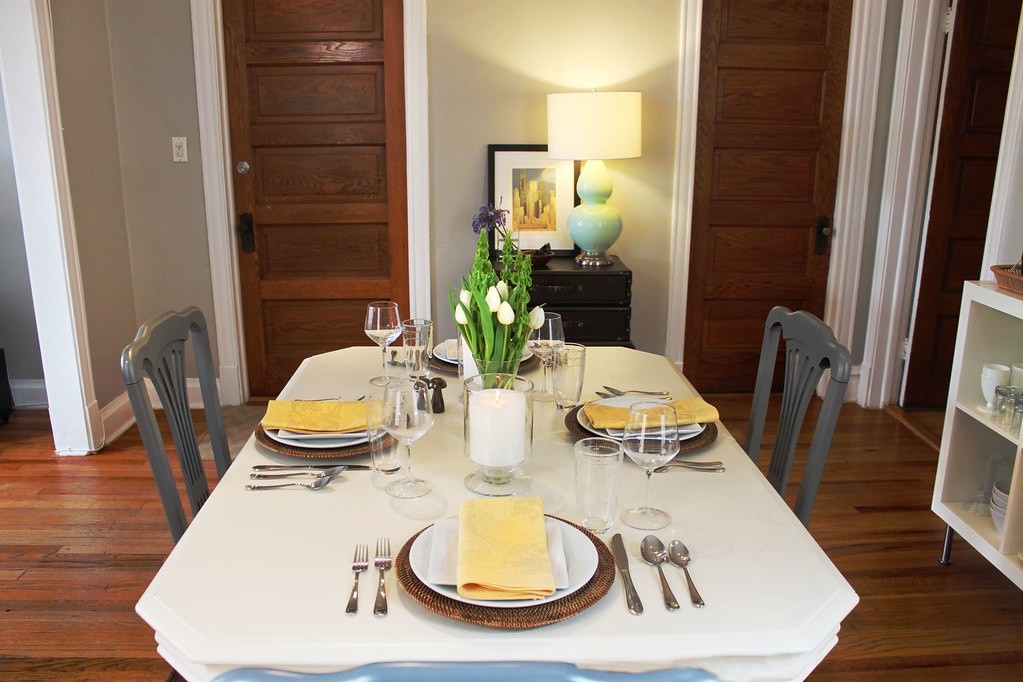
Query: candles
468,388,526,469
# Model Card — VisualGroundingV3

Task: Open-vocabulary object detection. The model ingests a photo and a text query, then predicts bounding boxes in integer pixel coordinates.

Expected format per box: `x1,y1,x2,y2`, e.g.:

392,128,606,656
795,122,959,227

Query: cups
552,342,586,413
1012,363,1023,389
367,376,403,474
402,317,434,383
573,437,624,536
991,385,1023,439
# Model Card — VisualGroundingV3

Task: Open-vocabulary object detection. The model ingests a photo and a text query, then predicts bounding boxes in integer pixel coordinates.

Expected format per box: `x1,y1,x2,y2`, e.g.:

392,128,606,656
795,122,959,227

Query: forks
345,543,368,615
373,537,392,617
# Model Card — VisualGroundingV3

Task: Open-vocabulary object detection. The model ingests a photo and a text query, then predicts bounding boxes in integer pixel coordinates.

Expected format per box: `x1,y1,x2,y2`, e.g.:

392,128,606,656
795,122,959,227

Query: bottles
428,377,447,413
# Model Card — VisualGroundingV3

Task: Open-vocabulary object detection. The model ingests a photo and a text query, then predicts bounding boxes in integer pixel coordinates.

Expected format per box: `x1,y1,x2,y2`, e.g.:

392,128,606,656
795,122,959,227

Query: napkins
583,395,724,428
455,497,554,604
258,394,392,431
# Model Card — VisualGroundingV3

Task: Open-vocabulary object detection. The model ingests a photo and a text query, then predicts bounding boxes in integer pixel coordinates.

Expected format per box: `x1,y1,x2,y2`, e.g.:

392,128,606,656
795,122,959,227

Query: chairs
745,306,852,521
121,305,232,542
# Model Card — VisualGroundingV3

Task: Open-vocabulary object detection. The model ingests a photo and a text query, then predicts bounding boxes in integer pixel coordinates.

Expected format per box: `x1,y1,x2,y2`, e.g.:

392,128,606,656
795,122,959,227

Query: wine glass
622,401,681,531
976,363,1011,414
527,311,565,403
381,378,436,499
364,301,403,387
462,372,535,497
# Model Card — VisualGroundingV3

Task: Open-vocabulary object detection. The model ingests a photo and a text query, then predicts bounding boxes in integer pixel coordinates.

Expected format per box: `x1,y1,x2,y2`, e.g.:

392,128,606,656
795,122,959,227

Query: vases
460,358,524,389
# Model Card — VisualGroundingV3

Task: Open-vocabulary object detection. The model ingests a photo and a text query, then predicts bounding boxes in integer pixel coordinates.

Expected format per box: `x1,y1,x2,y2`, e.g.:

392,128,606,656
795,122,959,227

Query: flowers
447,199,533,385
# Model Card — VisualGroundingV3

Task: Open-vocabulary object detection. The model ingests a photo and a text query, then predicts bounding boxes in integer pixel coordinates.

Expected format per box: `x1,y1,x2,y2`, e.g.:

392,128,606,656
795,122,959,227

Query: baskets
990,265,1023,295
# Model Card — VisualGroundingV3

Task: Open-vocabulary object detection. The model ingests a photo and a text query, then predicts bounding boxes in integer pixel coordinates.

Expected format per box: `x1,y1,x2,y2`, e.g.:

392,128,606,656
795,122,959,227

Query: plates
408,516,599,608
576,405,707,442
264,429,387,449
432,339,534,365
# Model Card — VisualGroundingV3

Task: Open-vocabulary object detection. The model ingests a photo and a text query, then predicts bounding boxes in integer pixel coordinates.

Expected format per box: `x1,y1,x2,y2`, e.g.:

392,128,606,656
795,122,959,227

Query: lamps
545,89,642,266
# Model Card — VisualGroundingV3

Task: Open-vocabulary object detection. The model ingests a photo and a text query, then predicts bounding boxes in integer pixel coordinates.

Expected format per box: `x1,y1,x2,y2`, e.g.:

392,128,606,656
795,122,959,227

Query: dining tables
136,346,860,682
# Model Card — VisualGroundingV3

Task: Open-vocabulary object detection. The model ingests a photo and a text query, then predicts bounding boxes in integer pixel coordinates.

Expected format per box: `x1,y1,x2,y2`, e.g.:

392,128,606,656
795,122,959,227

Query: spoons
594,386,672,402
668,539,705,608
244,474,333,491
250,466,346,478
640,535,680,611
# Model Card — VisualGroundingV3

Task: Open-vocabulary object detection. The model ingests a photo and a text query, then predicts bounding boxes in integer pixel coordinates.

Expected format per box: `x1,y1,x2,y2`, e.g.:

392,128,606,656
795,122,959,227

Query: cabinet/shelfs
928,280,1023,592
490,255,633,349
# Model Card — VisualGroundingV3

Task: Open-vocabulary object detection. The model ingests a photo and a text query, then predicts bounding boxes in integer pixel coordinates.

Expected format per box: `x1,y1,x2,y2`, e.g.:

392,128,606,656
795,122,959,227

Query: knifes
252,463,371,471
611,533,643,616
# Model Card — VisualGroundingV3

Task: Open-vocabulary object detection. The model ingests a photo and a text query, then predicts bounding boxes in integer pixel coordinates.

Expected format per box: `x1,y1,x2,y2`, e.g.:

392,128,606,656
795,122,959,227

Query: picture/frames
486,144,579,257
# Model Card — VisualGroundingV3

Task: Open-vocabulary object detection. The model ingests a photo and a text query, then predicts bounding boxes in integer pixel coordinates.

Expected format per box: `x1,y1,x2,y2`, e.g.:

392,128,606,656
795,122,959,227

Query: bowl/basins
522,250,555,267
989,478,1011,534
